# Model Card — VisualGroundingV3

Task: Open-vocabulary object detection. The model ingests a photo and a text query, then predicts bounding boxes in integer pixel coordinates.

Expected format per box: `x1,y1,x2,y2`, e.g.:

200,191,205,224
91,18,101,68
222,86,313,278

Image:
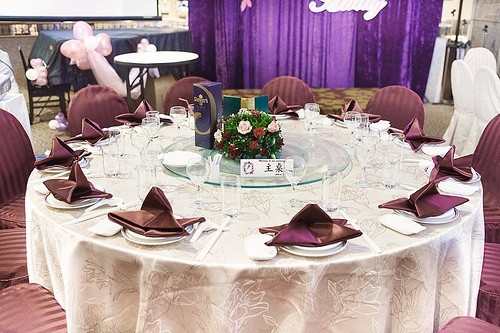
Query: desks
28,25,192,115
112,50,200,109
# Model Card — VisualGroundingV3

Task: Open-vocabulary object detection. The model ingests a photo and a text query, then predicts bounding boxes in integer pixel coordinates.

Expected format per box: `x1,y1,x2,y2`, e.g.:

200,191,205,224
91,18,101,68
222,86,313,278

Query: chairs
440,45,500,159
0,76,500,333
17,47,70,125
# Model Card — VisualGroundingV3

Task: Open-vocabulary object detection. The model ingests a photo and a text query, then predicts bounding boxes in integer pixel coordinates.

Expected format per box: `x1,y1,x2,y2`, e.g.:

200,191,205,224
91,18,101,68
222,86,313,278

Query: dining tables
423,35,468,103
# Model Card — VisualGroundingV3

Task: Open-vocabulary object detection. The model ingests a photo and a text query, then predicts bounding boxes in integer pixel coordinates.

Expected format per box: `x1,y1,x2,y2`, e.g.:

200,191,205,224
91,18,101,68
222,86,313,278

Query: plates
423,163,482,184
272,231,348,258
121,213,195,245
75,129,121,146
44,191,104,210
392,202,460,224
40,157,90,173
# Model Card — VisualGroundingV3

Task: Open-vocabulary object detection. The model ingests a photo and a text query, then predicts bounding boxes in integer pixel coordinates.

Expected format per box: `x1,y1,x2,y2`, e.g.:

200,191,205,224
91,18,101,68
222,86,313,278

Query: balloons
25,58,48,86
48,112,69,132
123,38,160,100
59,20,127,98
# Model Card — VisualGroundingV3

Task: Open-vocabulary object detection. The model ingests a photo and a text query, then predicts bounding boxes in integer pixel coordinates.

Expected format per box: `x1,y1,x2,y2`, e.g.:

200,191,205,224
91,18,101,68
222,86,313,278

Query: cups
321,169,342,211
354,114,369,141
387,133,405,164
102,143,120,177
136,163,156,200
146,110,161,123
381,150,402,189
108,128,126,157
305,102,320,132
188,104,197,131
221,174,242,217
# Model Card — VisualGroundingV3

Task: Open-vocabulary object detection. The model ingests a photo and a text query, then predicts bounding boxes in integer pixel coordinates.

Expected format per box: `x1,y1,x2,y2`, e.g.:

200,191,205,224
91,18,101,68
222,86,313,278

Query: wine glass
170,105,186,140
344,110,361,148
353,126,381,188
284,156,306,208
131,117,160,170
187,158,210,208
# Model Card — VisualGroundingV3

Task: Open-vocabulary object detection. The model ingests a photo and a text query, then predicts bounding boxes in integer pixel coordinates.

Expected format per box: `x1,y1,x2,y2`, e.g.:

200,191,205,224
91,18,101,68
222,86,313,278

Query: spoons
190,222,213,243
